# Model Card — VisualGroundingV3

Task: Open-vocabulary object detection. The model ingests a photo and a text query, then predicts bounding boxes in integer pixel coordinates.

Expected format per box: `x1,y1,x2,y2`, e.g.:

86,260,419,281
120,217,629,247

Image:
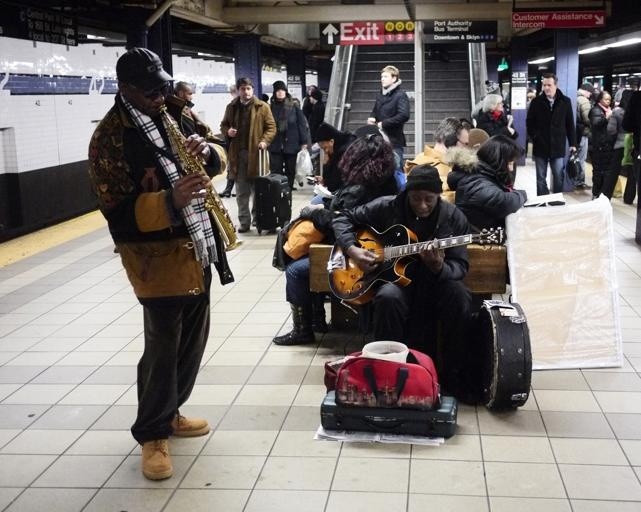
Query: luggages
251,149,292,235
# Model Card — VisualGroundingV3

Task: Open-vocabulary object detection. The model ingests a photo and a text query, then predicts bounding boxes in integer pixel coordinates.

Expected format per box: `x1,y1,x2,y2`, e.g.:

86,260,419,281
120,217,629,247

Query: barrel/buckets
361,339,410,364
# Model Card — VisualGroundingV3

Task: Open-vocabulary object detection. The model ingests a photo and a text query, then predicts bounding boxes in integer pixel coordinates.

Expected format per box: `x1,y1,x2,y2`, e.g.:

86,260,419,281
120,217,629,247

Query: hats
115,47,175,89
272,80,288,93
406,162,443,194
484,79,500,94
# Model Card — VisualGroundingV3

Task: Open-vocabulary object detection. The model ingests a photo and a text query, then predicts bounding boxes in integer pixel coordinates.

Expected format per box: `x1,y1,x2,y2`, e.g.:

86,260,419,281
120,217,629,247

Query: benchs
308,244,507,329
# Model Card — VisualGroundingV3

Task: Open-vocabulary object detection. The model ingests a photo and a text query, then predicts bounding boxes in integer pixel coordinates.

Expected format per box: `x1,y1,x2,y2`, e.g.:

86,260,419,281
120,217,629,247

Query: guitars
327,224,504,305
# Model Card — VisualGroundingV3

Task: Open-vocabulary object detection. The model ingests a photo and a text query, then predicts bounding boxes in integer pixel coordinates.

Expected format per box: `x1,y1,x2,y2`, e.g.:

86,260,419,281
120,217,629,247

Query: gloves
300,204,325,219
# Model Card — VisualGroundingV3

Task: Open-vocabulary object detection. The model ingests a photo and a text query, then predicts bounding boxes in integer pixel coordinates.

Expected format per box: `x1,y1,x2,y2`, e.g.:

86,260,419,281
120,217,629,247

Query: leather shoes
170,412,210,438
140,438,173,481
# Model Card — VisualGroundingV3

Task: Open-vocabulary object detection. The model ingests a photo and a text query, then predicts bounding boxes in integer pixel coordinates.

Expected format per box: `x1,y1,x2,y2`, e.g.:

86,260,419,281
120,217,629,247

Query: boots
271,301,329,346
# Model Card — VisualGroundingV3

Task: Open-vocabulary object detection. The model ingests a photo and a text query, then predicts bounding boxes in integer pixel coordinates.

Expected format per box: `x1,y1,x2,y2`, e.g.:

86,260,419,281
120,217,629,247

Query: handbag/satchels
294,148,314,183
333,339,442,410
272,216,324,272
564,147,587,190
620,134,635,166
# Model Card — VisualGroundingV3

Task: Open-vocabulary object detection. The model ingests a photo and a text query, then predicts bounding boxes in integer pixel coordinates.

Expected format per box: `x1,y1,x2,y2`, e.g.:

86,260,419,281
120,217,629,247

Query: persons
218,65,528,394
89,45,229,477
529,72,640,202
